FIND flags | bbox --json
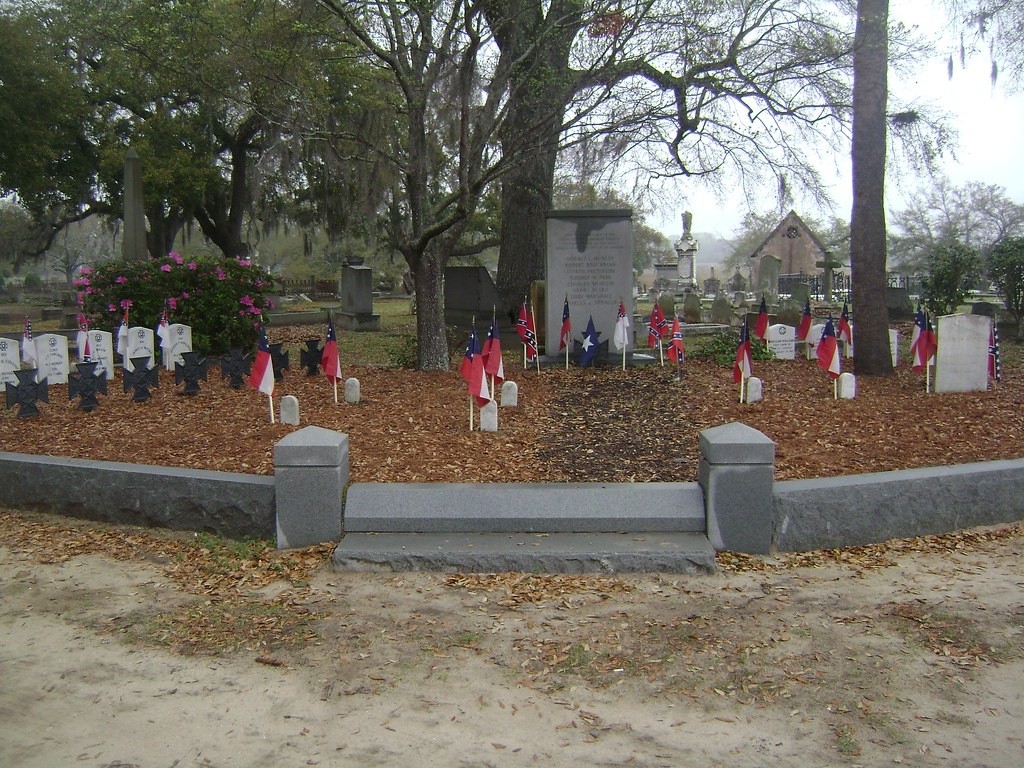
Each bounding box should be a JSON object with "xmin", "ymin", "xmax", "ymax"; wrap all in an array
[
  {"xmin": 527, "ymin": 311, "xmax": 539, "ymax": 364},
  {"xmin": 910, "ymin": 310, "xmax": 924, "ymax": 355},
  {"xmin": 116, "ymin": 307, "xmax": 131, "ymax": 357},
  {"xmin": 752, "ymin": 298, "xmax": 769, "ymax": 345},
  {"xmin": 249, "ymin": 324, "xmax": 276, "ymax": 398},
  {"xmin": 836, "ymin": 305, "xmax": 851, "ymax": 346},
  {"xmin": 482, "ymin": 315, "xmax": 505, "ymax": 385},
  {"xmin": 157, "ymin": 308, "xmax": 170, "ymax": 350},
  {"xmin": 559, "ymin": 299, "xmax": 572, "ymax": 351},
  {"xmin": 321, "ymin": 320, "xmax": 342, "ymax": 386},
  {"xmin": 816, "ymin": 319, "xmax": 840, "ymax": 379},
  {"xmin": 22, "ymin": 316, "xmax": 38, "ymax": 368},
  {"xmin": 77, "ymin": 309, "xmax": 93, "ymax": 363},
  {"xmin": 912, "ymin": 309, "xmax": 938, "ymax": 372},
  {"xmin": 613, "ymin": 302, "xmax": 630, "ymax": 351},
  {"xmin": 734, "ymin": 319, "xmax": 752, "ymax": 385},
  {"xmin": 988, "ymin": 327, "xmax": 999, "ymax": 380},
  {"xmin": 648, "ymin": 304, "xmax": 669, "ymax": 350},
  {"xmin": 580, "ymin": 316, "xmax": 600, "ymax": 367},
  {"xmin": 461, "ymin": 326, "xmax": 491, "ymax": 408},
  {"xmin": 667, "ymin": 317, "xmax": 685, "ymax": 364},
  {"xmin": 514, "ymin": 303, "xmax": 528, "ymax": 343},
  {"xmin": 797, "ymin": 301, "xmax": 814, "ymax": 348}
]
